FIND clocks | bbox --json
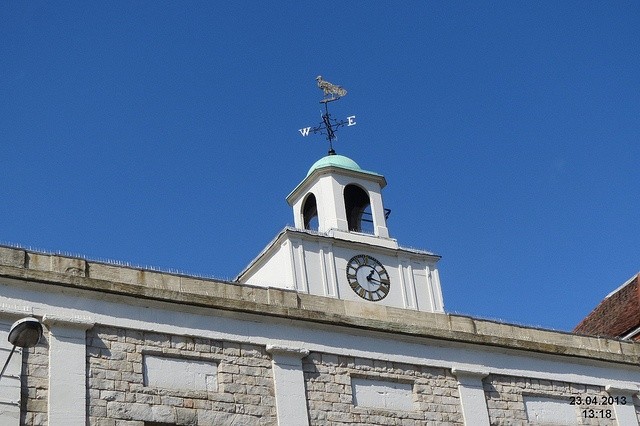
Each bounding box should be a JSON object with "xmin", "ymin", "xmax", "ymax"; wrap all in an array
[{"xmin": 346, "ymin": 254, "xmax": 390, "ymax": 302}]
